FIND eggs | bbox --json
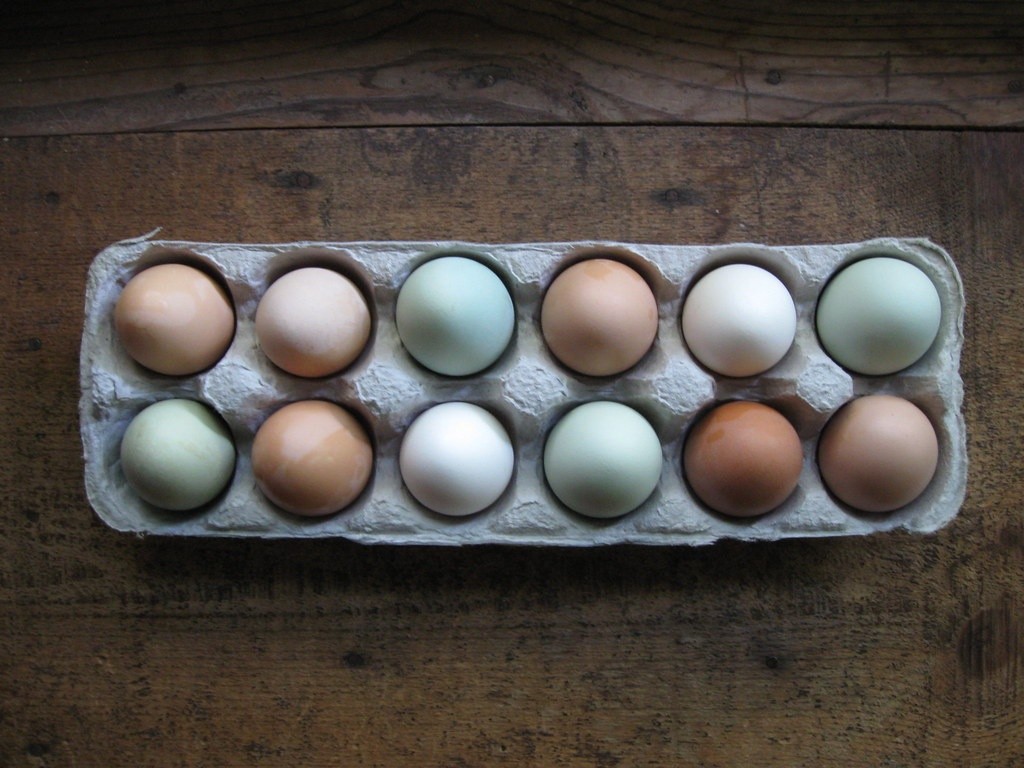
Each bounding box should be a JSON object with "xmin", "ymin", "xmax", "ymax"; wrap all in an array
[{"xmin": 112, "ymin": 255, "xmax": 943, "ymax": 519}]
